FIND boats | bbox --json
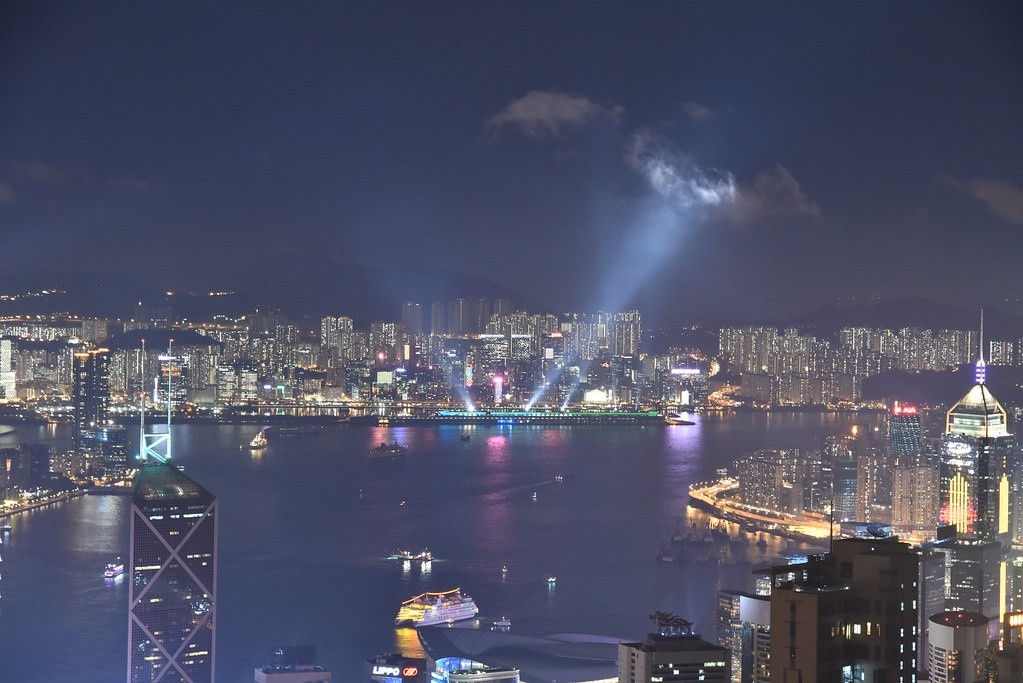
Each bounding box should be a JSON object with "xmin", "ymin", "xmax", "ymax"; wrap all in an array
[
  {"xmin": 105, "ymin": 564, "xmax": 125, "ymax": 578},
  {"xmin": 370, "ymin": 438, "xmax": 410, "ymax": 458},
  {"xmin": 430, "ymin": 655, "xmax": 522, "ymax": 683},
  {"xmin": 248, "ymin": 429, "xmax": 270, "ymax": 451},
  {"xmin": 396, "ymin": 586, "xmax": 480, "ymax": 626},
  {"xmin": 665, "ymin": 514, "xmax": 768, "ymax": 550}
]
